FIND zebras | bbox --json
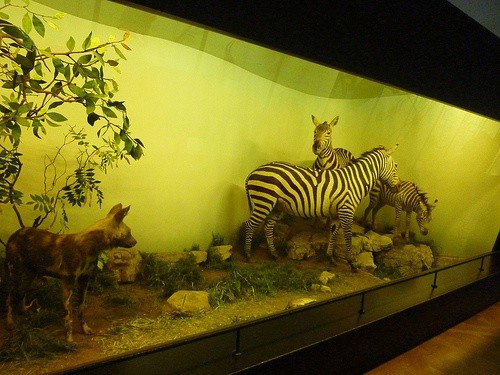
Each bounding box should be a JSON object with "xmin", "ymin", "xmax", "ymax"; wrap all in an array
[
  {"xmin": 239, "ymin": 143, "xmax": 402, "ymax": 274},
  {"xmin": 362, "ymin": 177, "xmax": 438, "ymax": 244}
]
